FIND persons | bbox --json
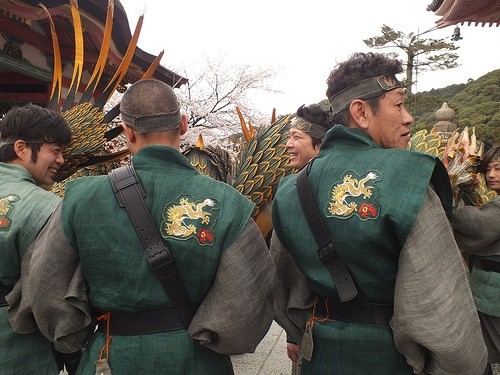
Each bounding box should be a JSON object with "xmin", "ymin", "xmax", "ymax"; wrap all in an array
[
  {"xmin": 257, "ymin": 104, "xmax": 339, "ymax": 375},
  {"xmin": 442, "ymin": 139, "xmax": 500, "ymax": 375},
  {"xmin": 30, "ymin": 79, "xmax": 278, "ymax": 375},
  {"xmin": 268, "ymin": 51, "xmax": 493, "ymax": 375},
  {"xmin": 1, "ymin": 102, "xmax": 71, "ymax": 375}
]
